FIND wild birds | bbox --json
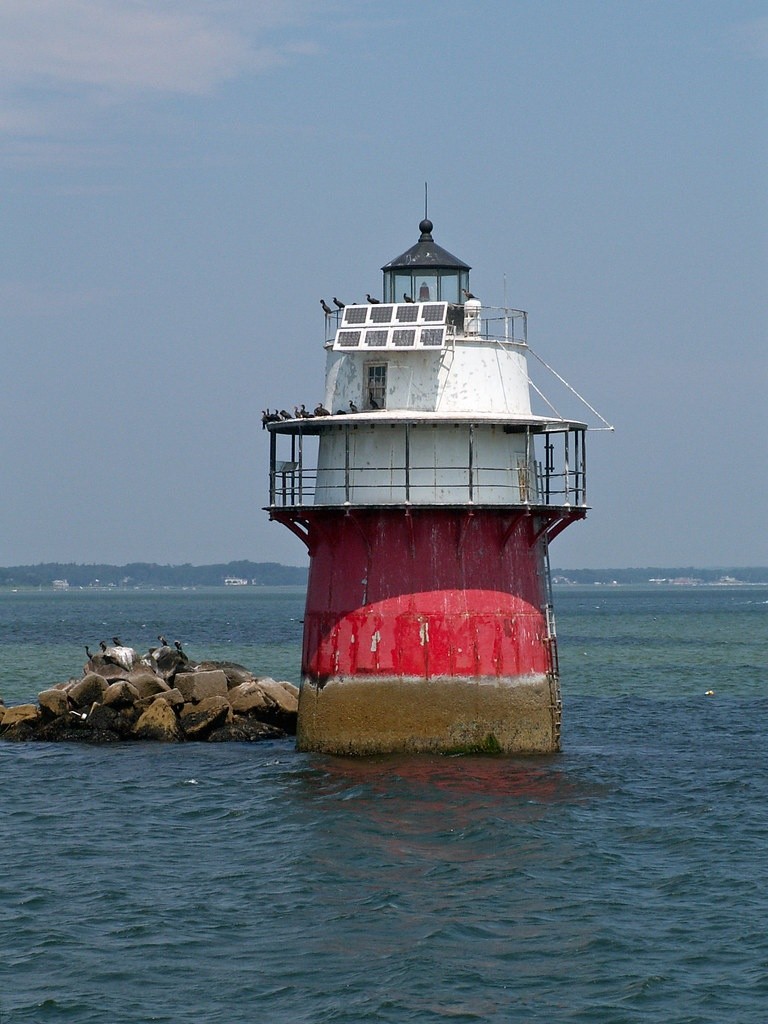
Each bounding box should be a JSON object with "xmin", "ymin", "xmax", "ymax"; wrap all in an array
[
  {"xmin": 461, "ymin": 288, "xmax": 480, "ymax": 301},
  {"xmin": 403, "ymin": 293, "xmax": 415, "ymax": 303},
  {"xmin": 84, "ymin": 633, "xmax": 186, "ymax": 674},
  {"xmin": 320, "ymin": 297, "xmax": 346, "ymax": 314},
  {"xmin": 365, "ymin": 293, "xmax": 380, "ymax": 304},
  {"xmin": 258, "ymin": 389, "xmax": 382, "ymax": 431}
]
